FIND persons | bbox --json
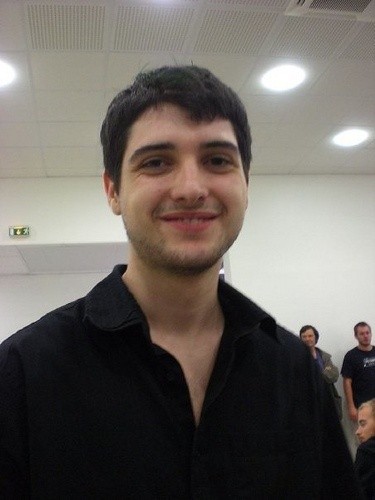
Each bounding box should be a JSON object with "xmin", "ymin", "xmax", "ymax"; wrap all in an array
[
  {"xmin": 0, "ymin": 65, "xmax": 358, "ymax": 500},
  {"xmin": 300, "ymin": 325, "xmax": 340, "ymax": 402},
  {"xmin": 354, "ymin": 398, "xmax": 375, "ymax": 500},
  {"xmin": 341, "ymin": 322, "xmax": 375, "ymax": 421}
]
